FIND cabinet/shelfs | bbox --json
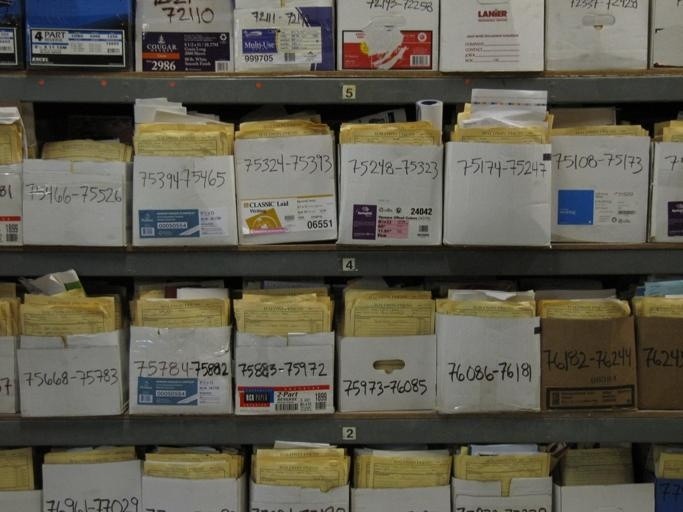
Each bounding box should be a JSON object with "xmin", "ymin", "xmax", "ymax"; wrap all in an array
[{"xmin": 0, "ymin": 72, "xmax": 682, "ymax": 447}]
